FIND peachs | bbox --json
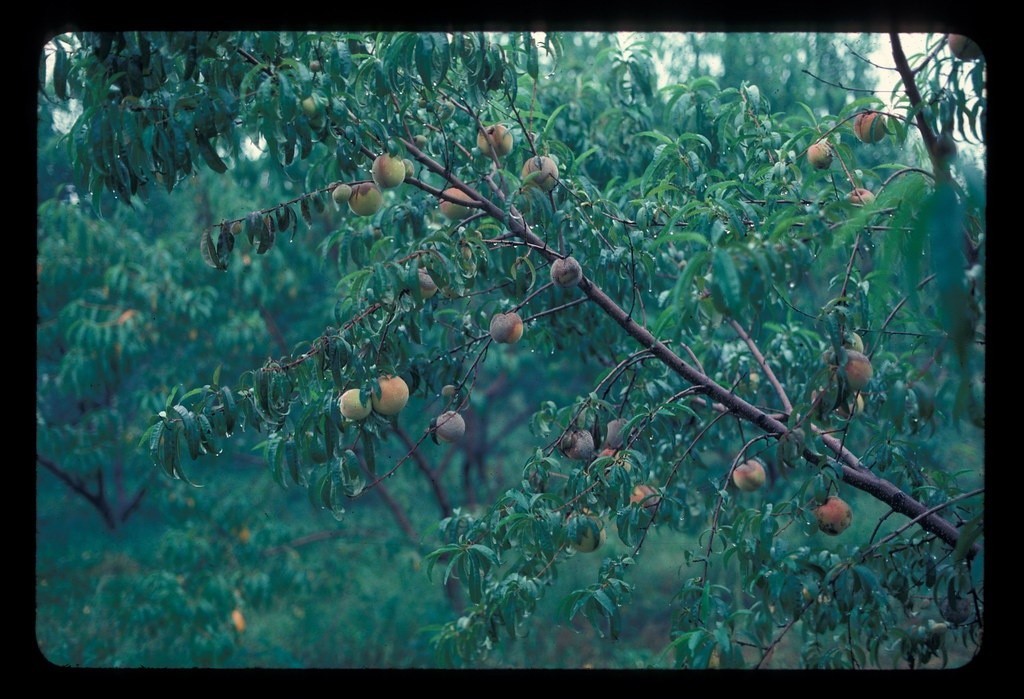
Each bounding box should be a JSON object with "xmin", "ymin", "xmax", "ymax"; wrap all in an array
[{"xmin": 302, "ymin": 33, "xmax": 978, "ymax": 668}]
